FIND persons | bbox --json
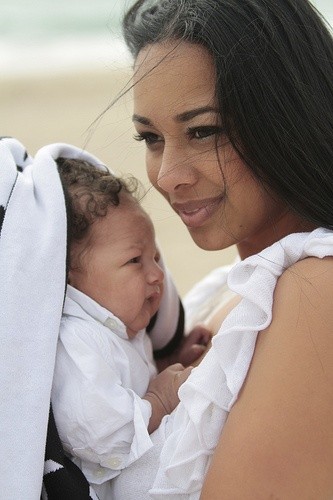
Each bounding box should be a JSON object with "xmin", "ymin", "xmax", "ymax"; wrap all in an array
[
  {"xmin": 0, "ymin": 138, "xmax": 216, "ymax": 499},
  {"xmin": 89, "ymin": 0, "xmax": 333, "ymax": 500}
]
